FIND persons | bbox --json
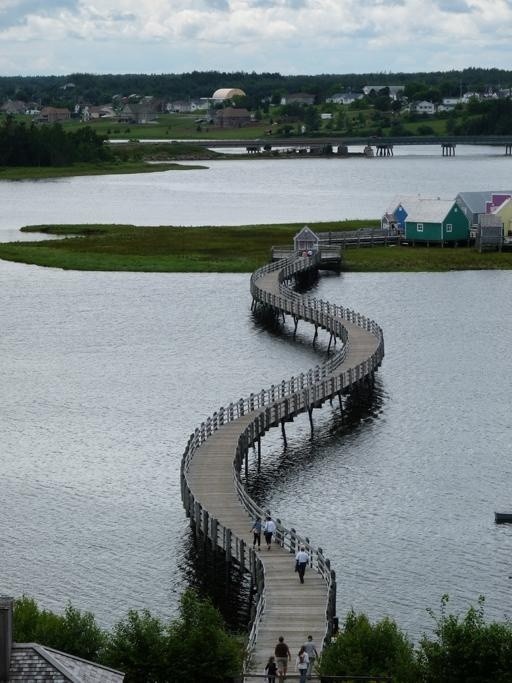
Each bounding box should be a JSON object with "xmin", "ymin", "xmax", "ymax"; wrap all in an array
[
  {"xmin": 296, "ymin": 645, "xmax": 309, "ymax": 683},
  {"xmin": 274, "ymin": 636, "xmax": 291, "ymax": 683},
  {"xmin": 250, "ymin": 517, "xmax": 264, "ymax": 551},
  {"xmin": 265, "ymin": 657, "xmax": 279, "ymax": 683},
  {"xmin": 303, "ymin": 635, "xmax": 317, "ymax": 680},
  {"xmin": 295, "ymin": 547, "xmax": 309, "ymax": 584},
  {"xmin": 263, "ymin": 517, "xmax": 276, "ymax": 551},
  {"xmin": 301, "ymin": 250, "xmax": 314, "ymax": 258}
]
